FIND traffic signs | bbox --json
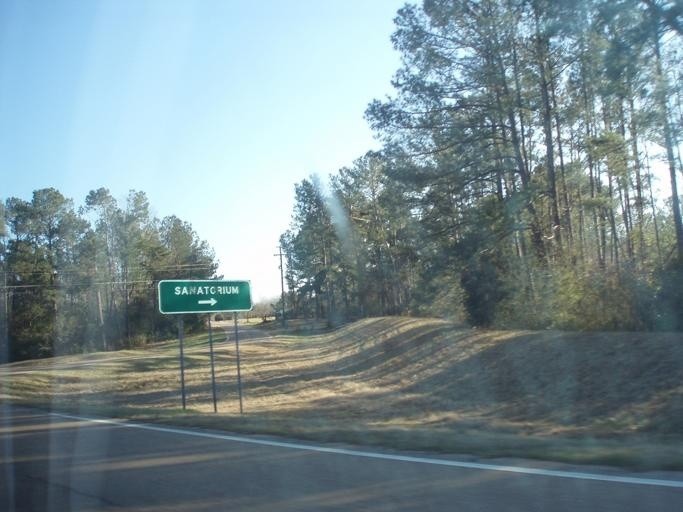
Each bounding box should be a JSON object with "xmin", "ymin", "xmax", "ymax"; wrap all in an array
[{"xmin": 157, "ymin": 279, "xmax": 252, "ymax": 314}]
